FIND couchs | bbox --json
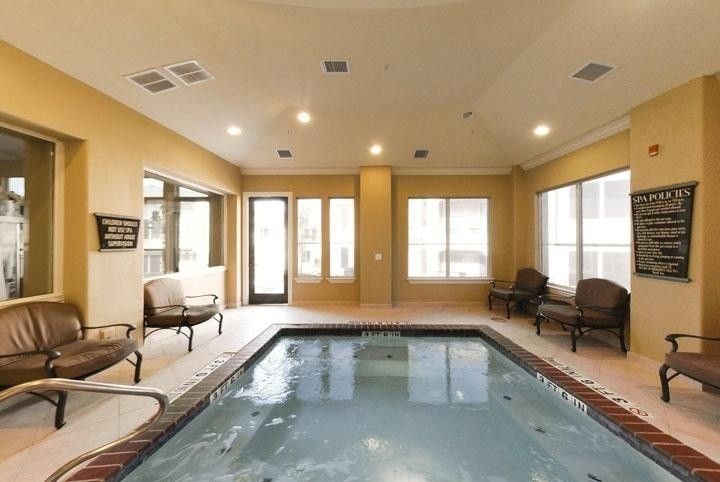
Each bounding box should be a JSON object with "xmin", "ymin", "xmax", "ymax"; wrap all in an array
[
  {"xmin": 145, "ymin": 276, "xmax": 224, "ymax": 352},
  {"xmin": 487, "ymin": 268, "xmax": 553, "ymax": 320},
  {"xmin": 658, "ymin": 333, "xmax": 720, "ymax": 403},
  {"xmin": 2, "ymin": 298, "xmax": 143, "ymax": 431},
  {"xmin": 536, "ymin": 276, "xmax": 630, "ymax": 354}
]
